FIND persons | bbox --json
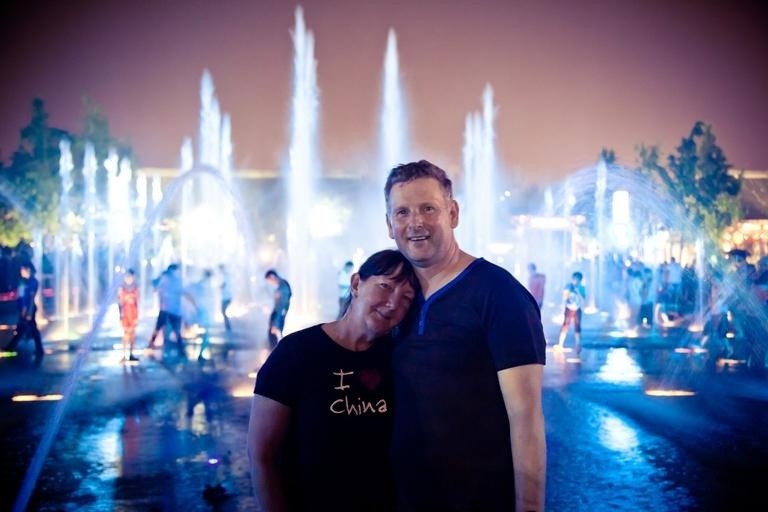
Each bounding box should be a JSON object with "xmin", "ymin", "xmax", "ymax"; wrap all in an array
[
  {"xmin": 577, "ymin": 250, "xmax": 768, "ymax": 372},
  {"xmin": 114, "ymin": 363, "xmax": 231, "ymax": 484},
  {"xmin": 265, "ymin": 270, "xmax": 293, "ymax": 347},
  {"xmin": 523, "ymin": 261, "xmax": 546, "ymax": 307},
  {"xmin": 148, "ymin": 262, "xmax": 232, "ymax": 362},
  {"xmin": 384, "ymin": 159, "xmax": 551, "ymax": 511},
  {"xmin": 117, "ymin": 268, "xmax": 140, "ymax": 362},
  {"xmin": 5, "ymin": 261, "xmax": 45, "ymax": 366},
  {"xmin": 558, "ymin": 272, "xmax": 585, "ymax": 356},
  {"xmin": 244, "ymin": 249, "xmax": 425, "ymax": 512},
  {"xmin": 335, "ymin": 260, "xmax": 354, "ymax": 318}
]
